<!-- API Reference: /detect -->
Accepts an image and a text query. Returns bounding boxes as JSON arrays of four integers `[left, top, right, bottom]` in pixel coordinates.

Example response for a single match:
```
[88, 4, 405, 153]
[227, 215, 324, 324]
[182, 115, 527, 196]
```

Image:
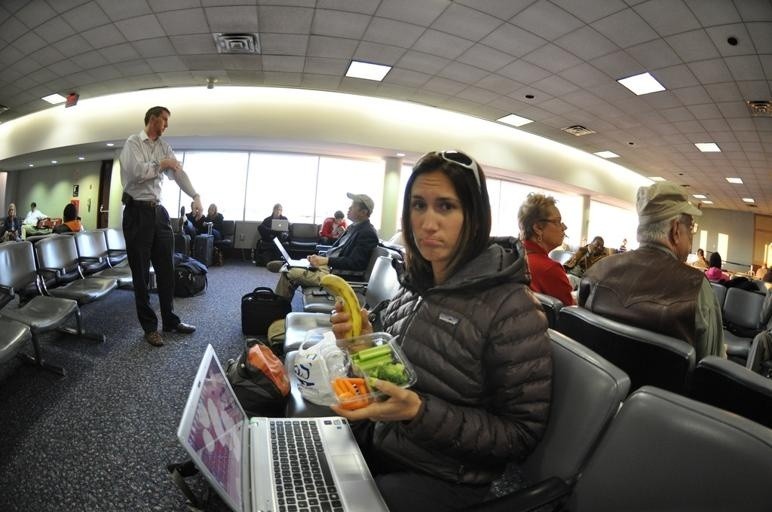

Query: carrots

[332, 376, 369, 408]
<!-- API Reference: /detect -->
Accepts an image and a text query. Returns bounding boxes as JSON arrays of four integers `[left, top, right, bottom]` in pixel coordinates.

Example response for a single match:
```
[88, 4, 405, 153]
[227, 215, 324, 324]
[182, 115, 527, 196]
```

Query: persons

[266, 192, 380, 303]
[514, 193, 577, 306]
[21, 201, 49, 240]
[51, 203, 82, 234]
[178, 201, 205, 247]
[332, 147, 555, 511]
[318, 210, 348, 247]
[202, 203, 225, 266]
[2, 202, 23, 242]
[257, 202, 293, 246]
[119, 105, 205, 347]
[690, 248, 729, 287]
[563, 235, 629, 279]
[577, 180, 729, 364]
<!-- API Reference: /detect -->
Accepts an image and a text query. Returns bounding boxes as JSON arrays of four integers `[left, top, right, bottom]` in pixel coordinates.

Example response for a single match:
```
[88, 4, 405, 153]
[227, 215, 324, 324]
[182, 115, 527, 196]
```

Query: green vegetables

[352, 342, 393, 369]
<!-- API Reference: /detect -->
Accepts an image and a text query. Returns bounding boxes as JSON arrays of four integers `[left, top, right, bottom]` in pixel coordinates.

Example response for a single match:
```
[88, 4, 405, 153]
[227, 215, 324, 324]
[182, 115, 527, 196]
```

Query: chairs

[218, 220, 233, 264]
[75, 228, 134, 292]
[290, 222, 317, 258]
[1, 240, 81, 381]
[38, 231, 118, 345]
[1, 281, 33, 392]
[109, 227, 154, 279]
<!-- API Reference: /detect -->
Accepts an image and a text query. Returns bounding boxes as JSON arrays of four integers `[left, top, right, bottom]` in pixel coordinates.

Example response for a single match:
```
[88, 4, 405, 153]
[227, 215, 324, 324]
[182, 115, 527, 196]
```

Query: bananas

[320, 274, 362, 339]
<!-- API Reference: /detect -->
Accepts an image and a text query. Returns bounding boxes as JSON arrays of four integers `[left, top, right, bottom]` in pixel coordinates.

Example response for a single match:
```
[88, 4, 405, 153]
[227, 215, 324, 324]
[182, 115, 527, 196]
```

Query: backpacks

[172, 252, 209, 299]
[224, 338, 292, 416]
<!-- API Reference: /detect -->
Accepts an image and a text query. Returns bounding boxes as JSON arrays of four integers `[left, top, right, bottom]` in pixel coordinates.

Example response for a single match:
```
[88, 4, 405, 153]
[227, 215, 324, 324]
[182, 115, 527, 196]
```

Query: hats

[636, 180, 703, 225]
[8, 203, 15, 210]
[347, 192, 374, 213]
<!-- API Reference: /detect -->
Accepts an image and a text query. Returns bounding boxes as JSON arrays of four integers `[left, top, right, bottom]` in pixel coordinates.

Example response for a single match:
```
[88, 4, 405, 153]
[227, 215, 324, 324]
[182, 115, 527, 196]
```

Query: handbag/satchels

[255, 238, 265, 267]
[241, 285, 293, 337]
[37, 219, 48, 229]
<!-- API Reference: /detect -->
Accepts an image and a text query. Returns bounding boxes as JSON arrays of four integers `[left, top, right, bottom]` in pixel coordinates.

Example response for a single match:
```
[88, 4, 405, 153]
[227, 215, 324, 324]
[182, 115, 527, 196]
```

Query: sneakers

[281, 233, 288, 239]
[266, 260, 287, 273]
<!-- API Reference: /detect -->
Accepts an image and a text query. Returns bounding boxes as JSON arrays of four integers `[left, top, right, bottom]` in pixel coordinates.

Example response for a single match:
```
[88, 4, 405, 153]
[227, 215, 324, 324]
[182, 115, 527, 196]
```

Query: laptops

[177, 343, 391, 512]
[271, 219, 289, 233]
[272, 236, 320, 271]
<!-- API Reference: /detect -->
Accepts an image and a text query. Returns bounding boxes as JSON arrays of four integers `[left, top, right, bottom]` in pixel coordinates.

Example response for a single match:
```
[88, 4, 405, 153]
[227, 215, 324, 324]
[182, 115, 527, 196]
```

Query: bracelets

[191, 193, 201, 199]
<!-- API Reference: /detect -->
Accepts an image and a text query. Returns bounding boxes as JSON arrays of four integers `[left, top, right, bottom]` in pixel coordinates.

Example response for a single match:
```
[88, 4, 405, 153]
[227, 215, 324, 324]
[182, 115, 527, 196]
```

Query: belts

[126, 201, 156, 207]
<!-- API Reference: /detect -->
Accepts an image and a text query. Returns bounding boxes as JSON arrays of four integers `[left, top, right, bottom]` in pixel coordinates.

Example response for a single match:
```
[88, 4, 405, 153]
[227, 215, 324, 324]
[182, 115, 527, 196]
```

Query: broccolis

[375, 363, 407, 383]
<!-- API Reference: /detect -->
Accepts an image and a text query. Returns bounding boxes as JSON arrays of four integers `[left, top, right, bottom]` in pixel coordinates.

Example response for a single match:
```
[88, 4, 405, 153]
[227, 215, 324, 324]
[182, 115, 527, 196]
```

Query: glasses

[413, 149, 482, 195]
[679, 221, 698, 233]
[536, 219, 560, 226]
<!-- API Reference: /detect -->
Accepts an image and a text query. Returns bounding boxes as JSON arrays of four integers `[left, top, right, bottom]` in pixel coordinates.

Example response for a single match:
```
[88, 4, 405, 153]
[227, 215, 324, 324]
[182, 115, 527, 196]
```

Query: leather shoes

[145, 330, 164, 346]
[180, 206, 187, 223]
[162, 323, 197, 334]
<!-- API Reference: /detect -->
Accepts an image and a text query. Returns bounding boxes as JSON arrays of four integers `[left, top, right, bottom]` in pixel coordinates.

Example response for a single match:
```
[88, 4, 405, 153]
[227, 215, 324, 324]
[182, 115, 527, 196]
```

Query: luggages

[174, 221, 214, 267]
